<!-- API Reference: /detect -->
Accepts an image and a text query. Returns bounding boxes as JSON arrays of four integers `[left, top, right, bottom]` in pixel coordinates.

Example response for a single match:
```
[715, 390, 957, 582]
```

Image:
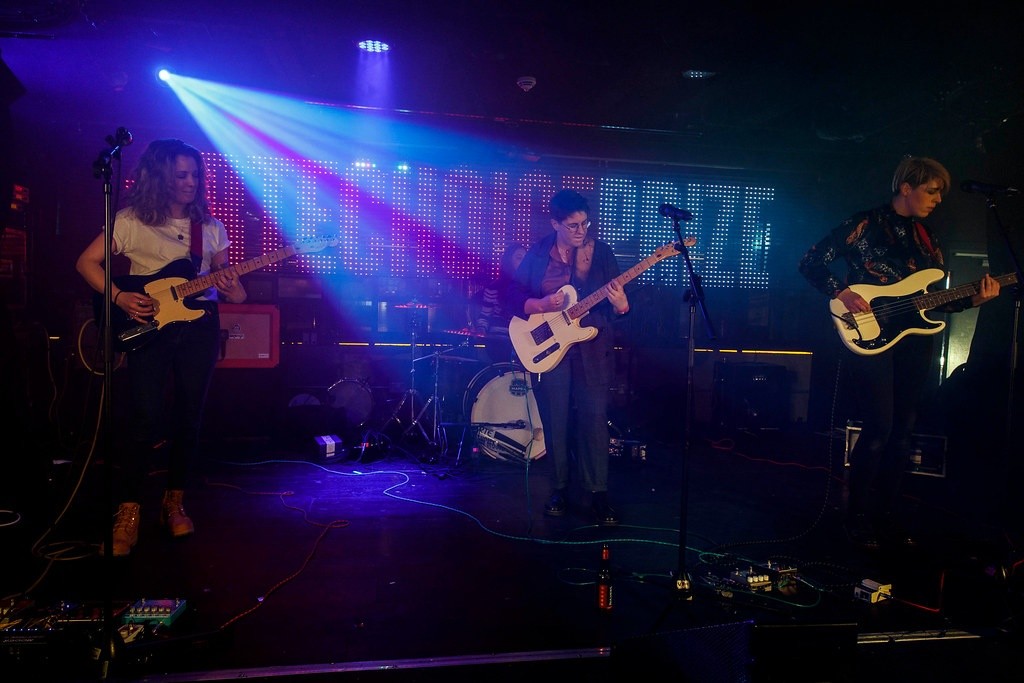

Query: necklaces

[167, 209, 188, 240]
[558, 241, 573, 254]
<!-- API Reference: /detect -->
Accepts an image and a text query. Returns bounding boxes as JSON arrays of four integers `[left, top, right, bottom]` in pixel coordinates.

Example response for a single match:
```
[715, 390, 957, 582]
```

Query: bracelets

[114, 290, 123, 304]
[617, 305, 629, 313]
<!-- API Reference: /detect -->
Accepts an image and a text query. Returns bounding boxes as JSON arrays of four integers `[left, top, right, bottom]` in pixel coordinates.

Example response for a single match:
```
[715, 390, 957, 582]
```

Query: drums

[461, 361, 547, 466]
[326, 376, 376, 425]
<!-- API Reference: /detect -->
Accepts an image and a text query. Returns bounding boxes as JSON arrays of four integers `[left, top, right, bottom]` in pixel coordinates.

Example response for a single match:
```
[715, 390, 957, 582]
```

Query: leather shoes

[601, 507, 619, 526]
[543, 490, 571, 517]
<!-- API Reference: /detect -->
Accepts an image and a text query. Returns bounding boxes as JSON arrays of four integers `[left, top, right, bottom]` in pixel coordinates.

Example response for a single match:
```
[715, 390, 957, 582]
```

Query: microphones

[116, 128, 132, 146]
[659, 204, 693, 221]
[220, 328, 229, 359]
[960, 179, 1020, 196]
[516, 420, 526, 429]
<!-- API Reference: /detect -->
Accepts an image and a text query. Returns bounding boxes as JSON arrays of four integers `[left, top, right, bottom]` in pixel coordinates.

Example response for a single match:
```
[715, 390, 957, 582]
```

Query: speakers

[215, 303, 281, 368]
[692, 348, 813, 426]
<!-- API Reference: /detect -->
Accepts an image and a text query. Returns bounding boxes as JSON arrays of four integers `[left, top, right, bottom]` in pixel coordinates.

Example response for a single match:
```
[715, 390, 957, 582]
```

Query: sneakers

[161, 489, 195, 537]
[100, 501, 141, 559]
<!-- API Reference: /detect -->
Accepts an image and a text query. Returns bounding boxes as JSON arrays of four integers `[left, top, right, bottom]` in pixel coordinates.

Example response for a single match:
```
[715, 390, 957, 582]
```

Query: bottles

[595, 543, 616, 612]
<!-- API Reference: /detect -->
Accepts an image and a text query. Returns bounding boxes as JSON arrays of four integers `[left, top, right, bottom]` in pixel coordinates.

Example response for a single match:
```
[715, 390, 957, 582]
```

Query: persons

[76, 137, 247, 557]
[799, 156, 1000, 549]
[475, 241, 528, 338]
[508, 187, 633, 529]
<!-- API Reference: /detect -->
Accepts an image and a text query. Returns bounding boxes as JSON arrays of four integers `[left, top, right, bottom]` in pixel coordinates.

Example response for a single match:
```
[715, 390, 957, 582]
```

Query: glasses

[561, 219, 592, 233]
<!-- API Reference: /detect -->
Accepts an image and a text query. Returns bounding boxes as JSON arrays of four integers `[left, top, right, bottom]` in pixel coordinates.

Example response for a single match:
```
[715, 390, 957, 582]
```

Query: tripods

[381, 307, 471, 456]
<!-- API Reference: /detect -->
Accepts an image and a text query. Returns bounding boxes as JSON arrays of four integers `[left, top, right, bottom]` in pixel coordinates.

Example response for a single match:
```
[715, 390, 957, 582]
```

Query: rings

[137, 299, 142, 305]
[134, 311, 138, 317]
[227, 284, 232, 288]
[131, 315, 134, 319]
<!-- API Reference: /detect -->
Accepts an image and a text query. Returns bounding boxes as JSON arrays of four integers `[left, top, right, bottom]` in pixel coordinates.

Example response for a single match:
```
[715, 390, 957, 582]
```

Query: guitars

[508, 234, 696, 375]
[826, 267, 1024, 358]
[93, 233, 339, 356]
